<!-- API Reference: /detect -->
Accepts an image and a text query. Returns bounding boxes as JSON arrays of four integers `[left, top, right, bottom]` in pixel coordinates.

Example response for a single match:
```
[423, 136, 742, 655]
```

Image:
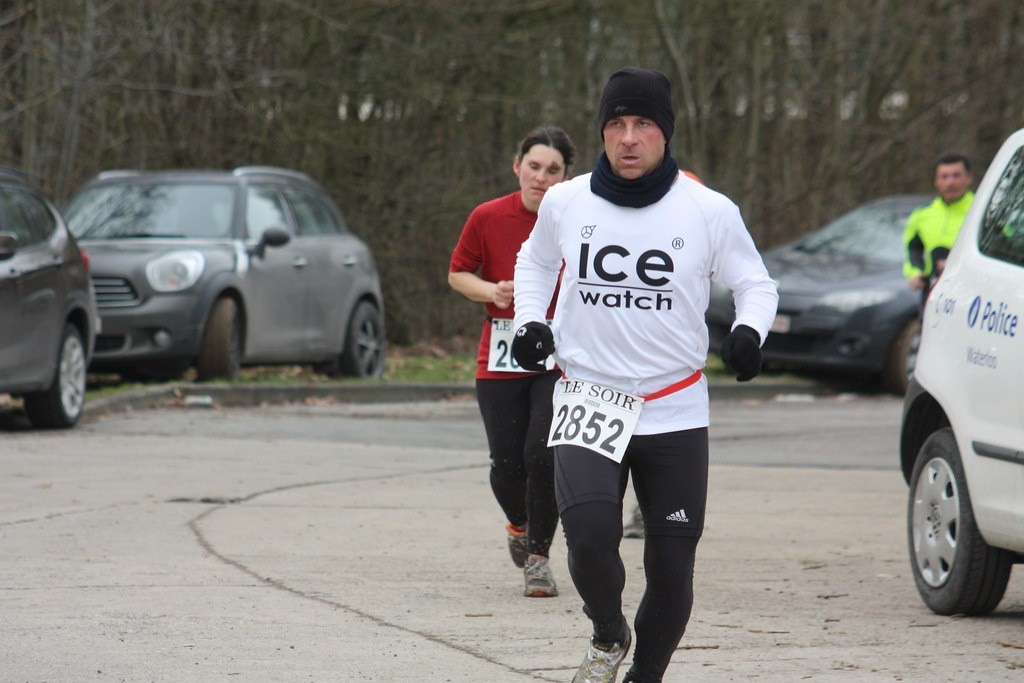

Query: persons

[513, 67, 780, 683]
[448, 125, 565, 598]
[620, 496, 647, 538]
[902, 152, 977, 314]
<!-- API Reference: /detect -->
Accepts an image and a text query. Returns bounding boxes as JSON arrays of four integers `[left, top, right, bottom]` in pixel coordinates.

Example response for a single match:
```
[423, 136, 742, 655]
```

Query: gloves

[718, 324, 762, 381]
[510, 321, 555, 372]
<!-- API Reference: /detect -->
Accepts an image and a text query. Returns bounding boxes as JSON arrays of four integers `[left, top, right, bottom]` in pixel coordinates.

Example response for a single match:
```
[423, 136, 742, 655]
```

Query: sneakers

[504, 522, 530, 568]
[623, 506, 645, 537]
[572, 614, 632, 683]
[523, 551, 559, 596]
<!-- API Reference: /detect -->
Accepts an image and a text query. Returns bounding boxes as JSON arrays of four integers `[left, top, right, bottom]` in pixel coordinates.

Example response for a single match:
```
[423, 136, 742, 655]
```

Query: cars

[897, 130, 1023, 617]
[705, 194, 941, 396]
[70, 166, 388, 384]
[1, 174, 99, 429]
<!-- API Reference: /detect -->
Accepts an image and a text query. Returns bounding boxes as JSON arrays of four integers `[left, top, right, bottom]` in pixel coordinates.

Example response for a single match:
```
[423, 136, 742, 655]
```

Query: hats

[598, 67, 676, 145]
